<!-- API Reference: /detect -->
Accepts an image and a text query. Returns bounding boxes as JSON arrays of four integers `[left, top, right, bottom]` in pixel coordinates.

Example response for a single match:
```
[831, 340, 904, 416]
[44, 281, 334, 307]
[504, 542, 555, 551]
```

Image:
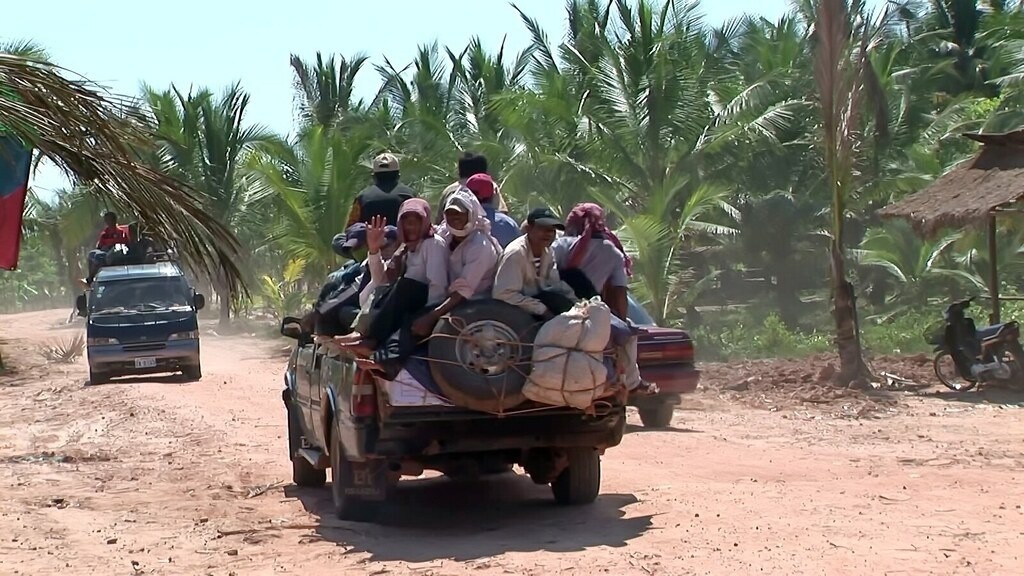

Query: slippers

[630, 380, 661, 397]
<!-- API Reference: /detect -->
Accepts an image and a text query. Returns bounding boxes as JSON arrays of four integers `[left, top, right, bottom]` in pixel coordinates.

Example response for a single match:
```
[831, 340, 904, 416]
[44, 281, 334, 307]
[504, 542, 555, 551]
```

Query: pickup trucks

[281, 261, 630, 521]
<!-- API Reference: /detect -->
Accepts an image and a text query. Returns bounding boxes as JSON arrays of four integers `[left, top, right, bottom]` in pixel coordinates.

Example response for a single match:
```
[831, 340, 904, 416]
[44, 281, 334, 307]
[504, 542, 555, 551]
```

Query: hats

[443, 203, 467, 213]
[342, 222, 367, 248]
[527, 208, 565, 231]
[373, 153, 400, 172]
[467, 172, 494, 199]
[380, 225, 397, 251]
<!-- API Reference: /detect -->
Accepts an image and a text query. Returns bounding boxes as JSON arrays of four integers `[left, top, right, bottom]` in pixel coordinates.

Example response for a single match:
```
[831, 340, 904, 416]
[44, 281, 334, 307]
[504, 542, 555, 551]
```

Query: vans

[76, 252, 205, 385]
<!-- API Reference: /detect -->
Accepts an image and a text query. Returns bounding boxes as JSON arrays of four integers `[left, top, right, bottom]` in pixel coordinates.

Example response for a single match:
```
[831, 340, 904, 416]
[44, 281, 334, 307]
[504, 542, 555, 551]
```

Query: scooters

[922, 295, 1024, 391]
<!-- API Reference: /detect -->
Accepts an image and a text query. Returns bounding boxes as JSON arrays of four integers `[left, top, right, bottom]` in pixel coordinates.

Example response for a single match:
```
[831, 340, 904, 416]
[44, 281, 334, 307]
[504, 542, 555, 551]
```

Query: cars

[625, 292, 702, 429]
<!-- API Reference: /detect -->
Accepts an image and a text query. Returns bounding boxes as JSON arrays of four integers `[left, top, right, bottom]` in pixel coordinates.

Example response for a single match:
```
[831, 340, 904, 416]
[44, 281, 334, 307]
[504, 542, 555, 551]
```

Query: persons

[341, 154, 418, 230]
[300, 219, 402, 343]
[158, 280, 186, 305]
[493, 207, 578, 323]
[339, 186, 506, 381]
[434, 153, 566, 249]
[551, 203, 661, 396]
[334, 194, 448, 345]
[86, 211, 130, 283]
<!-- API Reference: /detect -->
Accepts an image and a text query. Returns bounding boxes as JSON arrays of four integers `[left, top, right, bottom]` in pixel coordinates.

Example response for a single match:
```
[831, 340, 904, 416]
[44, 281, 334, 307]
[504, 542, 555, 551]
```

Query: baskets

[923, 318, 947, 345]
